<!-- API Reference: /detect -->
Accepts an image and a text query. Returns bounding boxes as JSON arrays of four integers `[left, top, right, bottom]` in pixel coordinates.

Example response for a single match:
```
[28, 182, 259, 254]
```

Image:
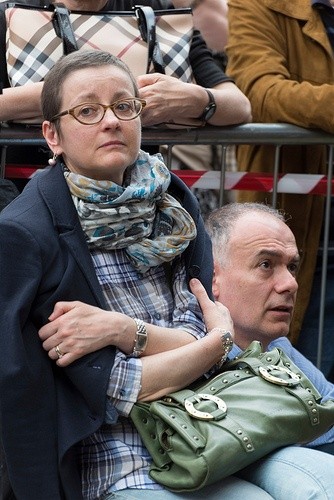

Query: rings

[56, 346, 63, 358]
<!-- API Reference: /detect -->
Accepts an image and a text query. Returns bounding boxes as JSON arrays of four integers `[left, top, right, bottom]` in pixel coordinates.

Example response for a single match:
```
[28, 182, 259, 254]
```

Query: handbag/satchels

[4, 2, 207, 129]
[132, 338, 334, 494]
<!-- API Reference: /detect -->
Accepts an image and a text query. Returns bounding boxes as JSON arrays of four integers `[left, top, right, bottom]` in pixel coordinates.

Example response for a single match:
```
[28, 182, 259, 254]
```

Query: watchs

[198, 90, 216, 121]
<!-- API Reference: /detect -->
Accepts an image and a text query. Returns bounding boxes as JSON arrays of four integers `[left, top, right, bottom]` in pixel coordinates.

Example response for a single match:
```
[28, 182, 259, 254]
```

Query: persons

[0, 51, 334, 500]
[205, 202, 334, 456]
[0, 0, 334, 385]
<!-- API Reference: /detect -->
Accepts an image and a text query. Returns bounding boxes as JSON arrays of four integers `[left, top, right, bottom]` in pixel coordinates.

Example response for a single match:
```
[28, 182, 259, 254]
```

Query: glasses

[49, 97, 146, 125]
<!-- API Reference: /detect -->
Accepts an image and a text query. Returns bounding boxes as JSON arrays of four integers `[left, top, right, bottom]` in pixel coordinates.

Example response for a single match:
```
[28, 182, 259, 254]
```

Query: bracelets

[206, 327, 233, 374]
[126, 318, 148, 358]
